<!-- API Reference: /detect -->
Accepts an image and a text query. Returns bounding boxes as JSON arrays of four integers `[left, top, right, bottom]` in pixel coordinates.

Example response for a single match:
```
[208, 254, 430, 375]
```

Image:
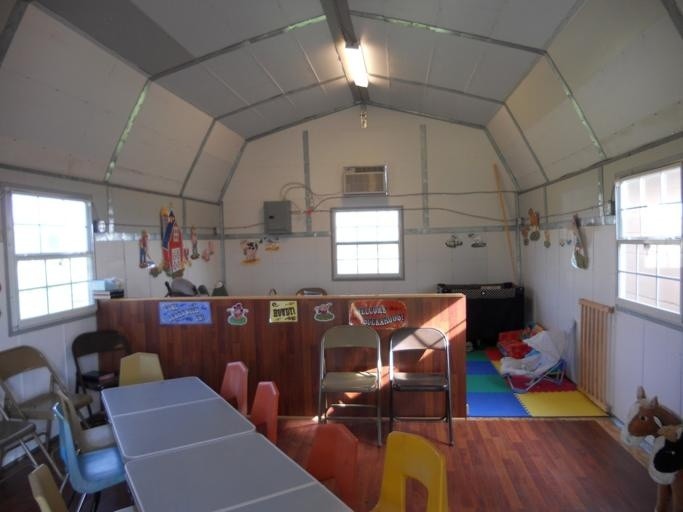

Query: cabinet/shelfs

[437, 282, 525, 349]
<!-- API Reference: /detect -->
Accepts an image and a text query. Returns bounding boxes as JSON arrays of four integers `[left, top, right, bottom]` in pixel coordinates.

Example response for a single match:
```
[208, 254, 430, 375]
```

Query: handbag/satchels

[571, 216, 587, 269]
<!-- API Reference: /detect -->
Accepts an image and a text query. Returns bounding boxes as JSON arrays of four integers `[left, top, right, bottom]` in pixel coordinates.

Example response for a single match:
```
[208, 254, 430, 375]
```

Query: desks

[124, 432, 355, 512]
[101, 375, 256, 461]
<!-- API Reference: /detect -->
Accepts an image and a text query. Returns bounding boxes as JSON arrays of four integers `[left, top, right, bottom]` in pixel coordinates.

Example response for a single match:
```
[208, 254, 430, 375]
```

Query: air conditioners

[343, 165, 388, 196]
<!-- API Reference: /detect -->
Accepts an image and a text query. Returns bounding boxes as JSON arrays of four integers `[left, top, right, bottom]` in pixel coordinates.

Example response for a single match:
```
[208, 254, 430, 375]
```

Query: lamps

[345, 43, 368, 88]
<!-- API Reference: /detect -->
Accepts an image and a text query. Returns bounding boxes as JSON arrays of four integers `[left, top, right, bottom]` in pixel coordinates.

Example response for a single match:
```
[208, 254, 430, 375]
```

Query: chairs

[28, 463, 140, 511]
[220, 360, 248, 415]
[57, 387, 115, 510]
[0, 345, 93, 480]
[119, 352, 164, 386]
[52, 402, 126, 512]
[369, 432, 449, 512]
[318, 324, 382, 447]
[0, 406, 38, 468]
[72, 330, 134, 427]
[250, 381, 279, 444]
[389, 327, 453, 446]
[306, 424, 359, 508]
